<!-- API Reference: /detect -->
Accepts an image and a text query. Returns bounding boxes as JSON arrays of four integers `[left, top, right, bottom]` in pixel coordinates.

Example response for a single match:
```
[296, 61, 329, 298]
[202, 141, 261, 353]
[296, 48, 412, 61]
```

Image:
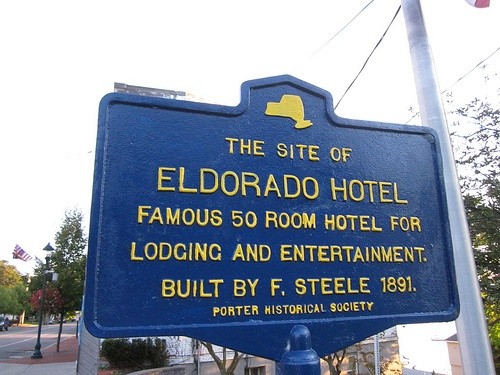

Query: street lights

[26, 242, 55, 361]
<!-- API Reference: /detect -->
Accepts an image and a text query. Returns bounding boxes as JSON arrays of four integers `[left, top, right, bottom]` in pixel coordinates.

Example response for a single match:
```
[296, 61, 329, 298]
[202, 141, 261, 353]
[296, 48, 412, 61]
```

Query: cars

[0, 316, 9, 332]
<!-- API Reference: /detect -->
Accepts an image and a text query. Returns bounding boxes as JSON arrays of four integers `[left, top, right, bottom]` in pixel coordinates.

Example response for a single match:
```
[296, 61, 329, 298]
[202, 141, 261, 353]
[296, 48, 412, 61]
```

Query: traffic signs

[80, 72, 462, 340]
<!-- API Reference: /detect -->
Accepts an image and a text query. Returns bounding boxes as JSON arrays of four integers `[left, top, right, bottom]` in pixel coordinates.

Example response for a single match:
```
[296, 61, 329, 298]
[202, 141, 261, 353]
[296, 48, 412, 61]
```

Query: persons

[3, 317, 9, 331]
